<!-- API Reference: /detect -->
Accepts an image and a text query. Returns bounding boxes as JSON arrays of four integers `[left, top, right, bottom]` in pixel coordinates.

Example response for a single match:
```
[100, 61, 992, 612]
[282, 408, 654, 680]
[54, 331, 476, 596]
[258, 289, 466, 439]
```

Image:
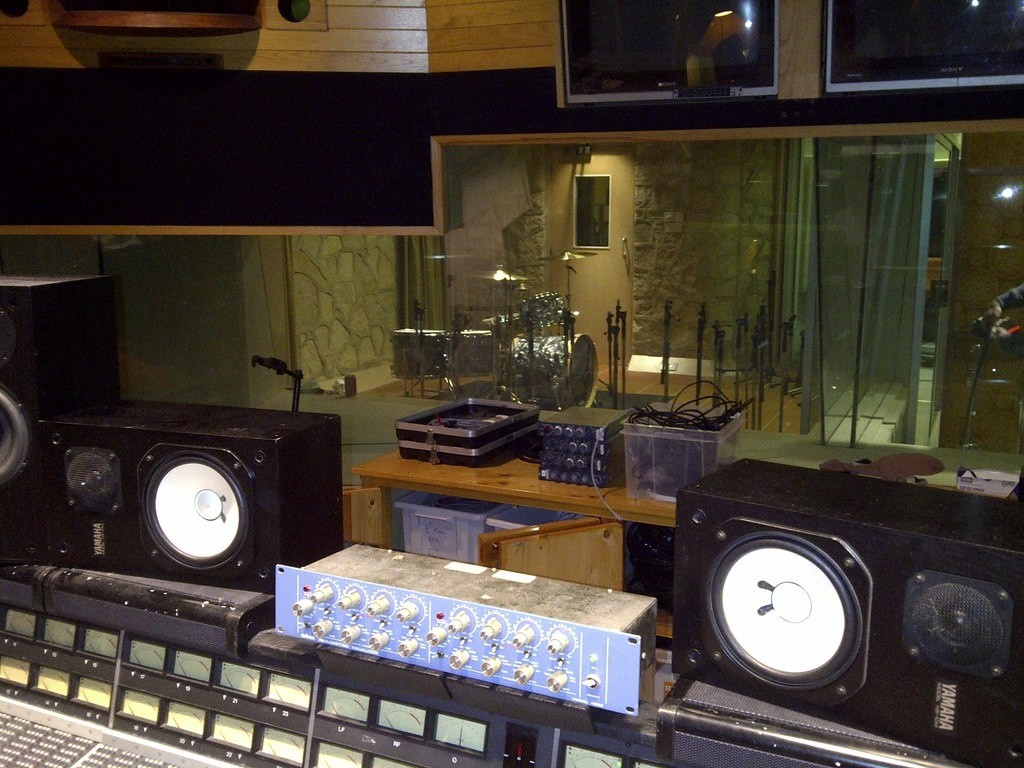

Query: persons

[981, 281, 1024, 359]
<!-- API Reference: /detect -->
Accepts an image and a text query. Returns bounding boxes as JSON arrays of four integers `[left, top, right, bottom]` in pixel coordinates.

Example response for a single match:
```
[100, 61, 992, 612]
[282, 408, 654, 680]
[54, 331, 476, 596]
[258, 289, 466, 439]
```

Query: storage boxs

[395, 397, 540, 467]
[393, 491, 512, 565]
[619, 401, 745, 502]
[485, 506, 584, 531]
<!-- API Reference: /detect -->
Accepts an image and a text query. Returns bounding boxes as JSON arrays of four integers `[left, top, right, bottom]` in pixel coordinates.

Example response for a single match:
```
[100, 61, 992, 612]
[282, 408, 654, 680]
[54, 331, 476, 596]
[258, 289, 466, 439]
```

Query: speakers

[0, 272, 342, 596]
[673, 458, 1024, 768]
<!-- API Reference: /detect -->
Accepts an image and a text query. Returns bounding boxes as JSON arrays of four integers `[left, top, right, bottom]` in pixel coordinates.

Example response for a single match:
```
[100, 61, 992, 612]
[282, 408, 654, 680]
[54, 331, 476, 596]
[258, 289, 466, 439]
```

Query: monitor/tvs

[557, 0, 779, 110]
[820, 0, 1024, 98]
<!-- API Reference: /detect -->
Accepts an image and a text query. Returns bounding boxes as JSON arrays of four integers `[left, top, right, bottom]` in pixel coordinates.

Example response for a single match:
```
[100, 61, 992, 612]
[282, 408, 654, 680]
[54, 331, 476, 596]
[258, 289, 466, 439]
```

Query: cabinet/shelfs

[342, 448, 677, 640]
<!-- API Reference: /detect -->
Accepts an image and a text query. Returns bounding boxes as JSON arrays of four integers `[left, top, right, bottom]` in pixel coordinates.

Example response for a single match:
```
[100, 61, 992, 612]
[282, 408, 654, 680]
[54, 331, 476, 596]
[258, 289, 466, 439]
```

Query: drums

[502, 331, 598, 412]
[450, 330, 493, 376]
[390, 328, 450, 380]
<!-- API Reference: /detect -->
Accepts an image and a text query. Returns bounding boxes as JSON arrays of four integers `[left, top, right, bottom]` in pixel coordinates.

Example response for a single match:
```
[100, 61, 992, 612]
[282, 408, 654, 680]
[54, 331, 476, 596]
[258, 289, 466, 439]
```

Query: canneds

[344, 373, 357, 397]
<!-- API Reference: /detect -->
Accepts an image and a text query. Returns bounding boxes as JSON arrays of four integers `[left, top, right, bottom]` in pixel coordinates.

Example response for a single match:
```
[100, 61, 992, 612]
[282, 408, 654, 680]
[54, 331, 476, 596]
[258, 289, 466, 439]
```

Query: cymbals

[481, 313, 520, 323]
[471, 269, 530, 282]
[538, 252, 598, 261]
